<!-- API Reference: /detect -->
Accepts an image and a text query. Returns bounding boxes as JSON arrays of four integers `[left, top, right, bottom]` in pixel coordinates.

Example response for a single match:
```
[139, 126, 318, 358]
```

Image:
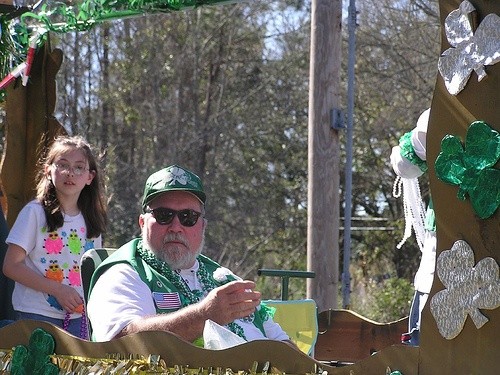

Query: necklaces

[63, 296, 87, 340]
[392, 175, 426, 253]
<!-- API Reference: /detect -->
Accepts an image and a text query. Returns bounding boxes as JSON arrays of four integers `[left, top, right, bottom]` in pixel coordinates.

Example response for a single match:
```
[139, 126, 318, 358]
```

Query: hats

[141, 164, 206, 209]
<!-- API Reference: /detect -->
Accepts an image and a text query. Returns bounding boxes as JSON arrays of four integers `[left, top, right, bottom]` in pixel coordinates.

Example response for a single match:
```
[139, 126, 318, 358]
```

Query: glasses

[145, 207, 202, 227]
[52, 164, 90, 176]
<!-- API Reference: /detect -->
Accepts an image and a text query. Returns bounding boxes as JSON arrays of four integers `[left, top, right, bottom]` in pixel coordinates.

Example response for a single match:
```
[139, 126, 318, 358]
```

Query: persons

[86, 164, 299, 351]
[3, 134, 110, 343]
[389, 106, 430, 344]
